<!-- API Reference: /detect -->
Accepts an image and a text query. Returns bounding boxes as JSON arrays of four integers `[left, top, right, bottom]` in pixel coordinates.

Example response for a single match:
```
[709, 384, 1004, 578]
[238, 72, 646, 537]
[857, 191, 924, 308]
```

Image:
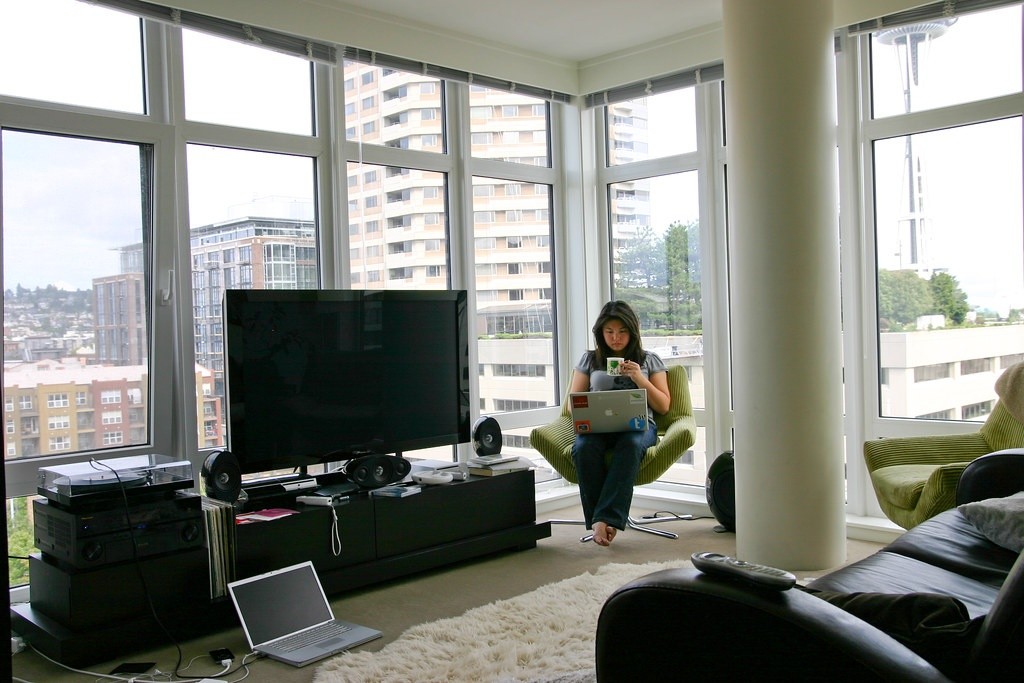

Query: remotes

[690, 550, 797, 590]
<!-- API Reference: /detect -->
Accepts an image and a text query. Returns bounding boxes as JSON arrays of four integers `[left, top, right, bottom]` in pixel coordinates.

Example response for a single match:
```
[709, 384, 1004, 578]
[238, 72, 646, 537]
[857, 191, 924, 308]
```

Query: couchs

[595, 447, 1024, 683]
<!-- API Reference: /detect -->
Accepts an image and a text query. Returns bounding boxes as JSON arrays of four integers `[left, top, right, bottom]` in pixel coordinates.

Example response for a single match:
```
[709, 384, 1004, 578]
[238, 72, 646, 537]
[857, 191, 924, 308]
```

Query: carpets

[312, 557, 697, 683]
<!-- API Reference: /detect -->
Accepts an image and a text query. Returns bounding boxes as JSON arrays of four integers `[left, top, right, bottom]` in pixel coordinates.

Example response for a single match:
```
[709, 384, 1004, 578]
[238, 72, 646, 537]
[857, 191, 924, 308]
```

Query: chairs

[861, 362, 1024, 530]
[531, 364, 695, 543]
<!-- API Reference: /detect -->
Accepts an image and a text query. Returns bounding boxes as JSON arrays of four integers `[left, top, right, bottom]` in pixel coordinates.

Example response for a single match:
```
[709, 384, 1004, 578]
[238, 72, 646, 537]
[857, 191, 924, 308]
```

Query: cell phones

[209, 648, 236, 664]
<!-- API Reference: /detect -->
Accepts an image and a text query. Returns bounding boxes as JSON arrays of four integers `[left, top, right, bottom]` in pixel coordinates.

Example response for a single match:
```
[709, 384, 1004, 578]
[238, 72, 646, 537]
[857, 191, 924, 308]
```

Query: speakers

[201, 450, 241, 504]
[472, 415, 503, 458]
[344, 455, 411, 490]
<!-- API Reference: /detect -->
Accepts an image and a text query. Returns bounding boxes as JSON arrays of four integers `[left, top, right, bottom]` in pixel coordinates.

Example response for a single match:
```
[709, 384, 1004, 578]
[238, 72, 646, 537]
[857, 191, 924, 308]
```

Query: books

[368, 486, 421, 497]
[467, 454, 537, 476]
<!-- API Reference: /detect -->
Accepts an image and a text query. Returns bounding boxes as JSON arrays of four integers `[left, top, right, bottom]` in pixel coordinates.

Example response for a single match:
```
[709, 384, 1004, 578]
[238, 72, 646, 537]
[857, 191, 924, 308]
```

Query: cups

[607, 357, 630, 376]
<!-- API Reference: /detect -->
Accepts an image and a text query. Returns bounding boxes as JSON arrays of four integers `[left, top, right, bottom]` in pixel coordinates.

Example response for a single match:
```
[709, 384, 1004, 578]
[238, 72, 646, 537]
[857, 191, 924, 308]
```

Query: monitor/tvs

[222, 289, 471, 497]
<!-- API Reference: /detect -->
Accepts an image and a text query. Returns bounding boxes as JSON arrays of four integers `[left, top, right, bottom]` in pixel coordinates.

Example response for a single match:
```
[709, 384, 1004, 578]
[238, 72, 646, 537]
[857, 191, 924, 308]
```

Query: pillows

[958, 490, 1024, 554]
[809, 593, 969, 675]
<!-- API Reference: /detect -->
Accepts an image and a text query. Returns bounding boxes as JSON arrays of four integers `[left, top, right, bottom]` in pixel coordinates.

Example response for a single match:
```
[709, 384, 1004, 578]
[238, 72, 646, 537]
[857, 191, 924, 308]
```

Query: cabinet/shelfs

[10, 465, 550, 670]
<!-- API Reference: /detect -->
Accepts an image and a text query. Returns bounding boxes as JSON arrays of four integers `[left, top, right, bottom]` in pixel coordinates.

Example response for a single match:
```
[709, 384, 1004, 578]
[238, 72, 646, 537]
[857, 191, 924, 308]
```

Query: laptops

[229, 560, 383, 669]
[569, 388, 649, 434]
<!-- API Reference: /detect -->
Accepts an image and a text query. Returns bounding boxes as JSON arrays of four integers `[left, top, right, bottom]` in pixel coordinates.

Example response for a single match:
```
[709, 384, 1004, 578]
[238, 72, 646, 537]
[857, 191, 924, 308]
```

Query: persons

[567, 300, 671, 546]
